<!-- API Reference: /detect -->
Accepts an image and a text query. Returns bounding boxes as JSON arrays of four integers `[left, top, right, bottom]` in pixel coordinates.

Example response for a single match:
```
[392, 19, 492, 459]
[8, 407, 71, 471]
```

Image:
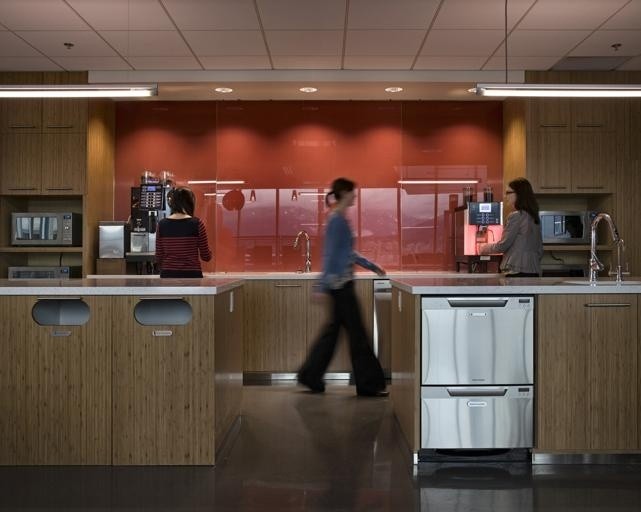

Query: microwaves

[539, 211, 600, 244]
[8, 265, 82, 280]
[11, 212, 81, 245]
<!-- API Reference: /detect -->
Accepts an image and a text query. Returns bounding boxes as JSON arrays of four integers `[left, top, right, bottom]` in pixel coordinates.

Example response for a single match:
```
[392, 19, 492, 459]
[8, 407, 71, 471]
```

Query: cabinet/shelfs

[1, 72, 118, 277]
[534, 295, 641, 458]
[3, 288, 244, 464]
[246, 280, 374, 372]
[501, 67, 641, 273]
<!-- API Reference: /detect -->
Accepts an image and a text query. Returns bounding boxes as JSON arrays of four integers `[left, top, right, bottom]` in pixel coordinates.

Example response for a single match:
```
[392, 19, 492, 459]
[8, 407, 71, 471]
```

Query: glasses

[504, 191, 516, 195]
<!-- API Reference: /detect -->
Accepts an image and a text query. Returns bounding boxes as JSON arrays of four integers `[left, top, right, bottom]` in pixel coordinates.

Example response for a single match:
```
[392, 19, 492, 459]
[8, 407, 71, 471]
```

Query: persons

[153, 186, 212, 278]
[297, 177, 393, 397]
[477, 177, 544, 278]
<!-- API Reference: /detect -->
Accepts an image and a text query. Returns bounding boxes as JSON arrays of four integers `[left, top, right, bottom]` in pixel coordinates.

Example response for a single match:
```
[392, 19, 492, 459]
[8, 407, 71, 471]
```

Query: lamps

[477, 0, 641, 104]
[290, 191, 297, 202]
[1, 1, 157, 102]
[248, 188, 257, 202]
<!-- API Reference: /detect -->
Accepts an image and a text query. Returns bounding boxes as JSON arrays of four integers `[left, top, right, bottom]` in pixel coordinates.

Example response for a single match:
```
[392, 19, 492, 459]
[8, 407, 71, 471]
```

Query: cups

[139, 169, 154, 184]
[157, 170, 176, 186]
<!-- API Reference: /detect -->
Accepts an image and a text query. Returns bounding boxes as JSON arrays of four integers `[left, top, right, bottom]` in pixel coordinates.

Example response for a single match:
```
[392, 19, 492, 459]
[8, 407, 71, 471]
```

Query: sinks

[562, 279, 641, 288]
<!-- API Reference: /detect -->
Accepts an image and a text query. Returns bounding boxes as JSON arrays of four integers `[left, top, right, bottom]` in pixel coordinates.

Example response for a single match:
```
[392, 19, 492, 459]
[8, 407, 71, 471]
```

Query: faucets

[292, 229, 312, 272]
[587, 208, 619, 281]
[617, 237, 625, 281]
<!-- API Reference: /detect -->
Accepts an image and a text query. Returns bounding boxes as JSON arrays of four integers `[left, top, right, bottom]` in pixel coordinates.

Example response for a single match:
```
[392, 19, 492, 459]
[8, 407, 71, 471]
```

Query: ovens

[419, 297, 532, 450]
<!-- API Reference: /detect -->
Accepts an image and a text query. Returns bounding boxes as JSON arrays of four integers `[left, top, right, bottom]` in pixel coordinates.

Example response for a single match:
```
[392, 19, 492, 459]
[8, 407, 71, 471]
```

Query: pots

[221, 188, 244, 211]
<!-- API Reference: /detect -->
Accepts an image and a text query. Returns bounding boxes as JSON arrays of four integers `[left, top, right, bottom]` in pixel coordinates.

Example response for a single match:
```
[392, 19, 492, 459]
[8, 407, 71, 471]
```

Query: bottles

[483, 183, 493, 202]
[448, 194, 458, 212]
[462, 183, 473, 205]
